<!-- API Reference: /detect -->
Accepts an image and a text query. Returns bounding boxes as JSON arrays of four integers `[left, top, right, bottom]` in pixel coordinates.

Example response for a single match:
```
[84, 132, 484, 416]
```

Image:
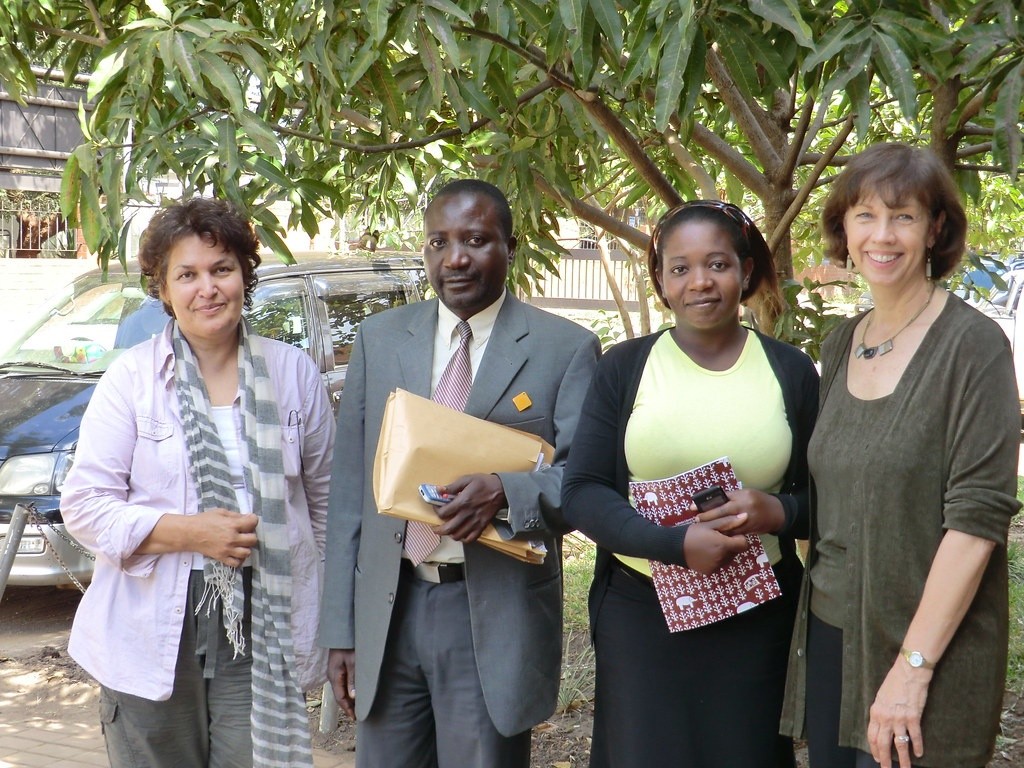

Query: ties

[403, 322, 474, 569]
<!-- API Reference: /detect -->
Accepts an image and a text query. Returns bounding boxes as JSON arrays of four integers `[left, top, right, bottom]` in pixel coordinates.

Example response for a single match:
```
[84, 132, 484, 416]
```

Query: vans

[1, 248, 433, 589]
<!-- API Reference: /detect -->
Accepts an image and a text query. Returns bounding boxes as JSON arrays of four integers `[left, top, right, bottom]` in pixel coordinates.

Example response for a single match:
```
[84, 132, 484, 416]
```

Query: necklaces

[854, 280, 936, 360]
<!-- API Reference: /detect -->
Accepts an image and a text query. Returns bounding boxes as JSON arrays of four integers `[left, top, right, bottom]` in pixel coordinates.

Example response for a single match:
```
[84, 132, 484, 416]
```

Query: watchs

[898, 646, 937, 670]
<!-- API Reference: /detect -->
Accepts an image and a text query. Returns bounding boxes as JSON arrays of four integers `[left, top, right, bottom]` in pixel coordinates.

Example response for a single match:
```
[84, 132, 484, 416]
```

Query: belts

[400, 558, 466, 585]
[608, 555, 805, 641]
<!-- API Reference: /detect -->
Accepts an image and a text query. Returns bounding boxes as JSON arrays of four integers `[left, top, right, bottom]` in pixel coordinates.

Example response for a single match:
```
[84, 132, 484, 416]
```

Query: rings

[893, 734, 910, 743]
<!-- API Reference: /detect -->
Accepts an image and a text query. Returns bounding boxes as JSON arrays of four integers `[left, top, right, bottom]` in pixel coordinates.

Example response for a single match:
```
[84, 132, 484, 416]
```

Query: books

[627, 456, 783, 634]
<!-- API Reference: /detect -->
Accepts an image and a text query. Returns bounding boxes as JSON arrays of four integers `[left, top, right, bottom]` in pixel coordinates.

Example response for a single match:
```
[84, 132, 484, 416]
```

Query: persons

[774, 142, 1023, 768]
[317, 177, 605, 768]
[559, 197, 826, 768]
[58, 197, 337, 768]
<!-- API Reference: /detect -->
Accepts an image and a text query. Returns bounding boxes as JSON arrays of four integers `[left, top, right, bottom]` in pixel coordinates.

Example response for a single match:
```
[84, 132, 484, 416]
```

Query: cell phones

[418, 484, 452, 506]
[691, 485, 730, 514]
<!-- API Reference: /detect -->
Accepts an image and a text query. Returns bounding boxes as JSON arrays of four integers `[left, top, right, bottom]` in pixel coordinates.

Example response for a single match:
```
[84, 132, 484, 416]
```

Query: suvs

[946, 251, 1024, 416]
